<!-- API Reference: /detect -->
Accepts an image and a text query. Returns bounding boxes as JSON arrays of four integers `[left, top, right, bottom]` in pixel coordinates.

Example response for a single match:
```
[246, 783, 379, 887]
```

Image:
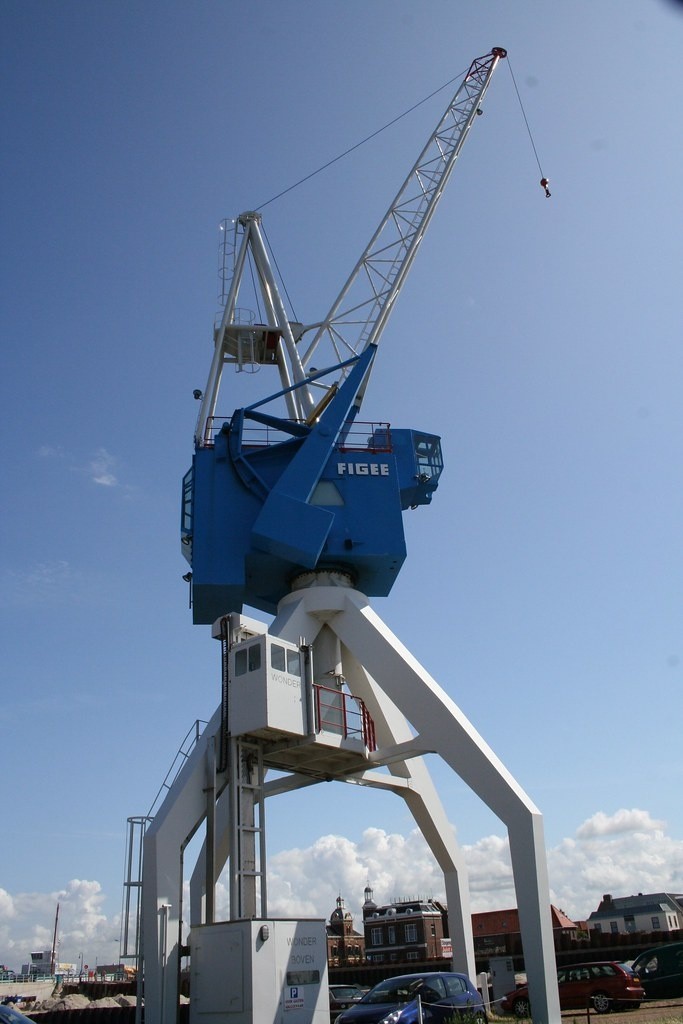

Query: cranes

[134, 47, 583, 1024]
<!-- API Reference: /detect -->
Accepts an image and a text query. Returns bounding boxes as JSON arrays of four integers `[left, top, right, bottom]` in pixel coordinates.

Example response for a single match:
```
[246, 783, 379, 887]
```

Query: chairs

[570, 972, 577, 980]
[580, 972, 588, 979]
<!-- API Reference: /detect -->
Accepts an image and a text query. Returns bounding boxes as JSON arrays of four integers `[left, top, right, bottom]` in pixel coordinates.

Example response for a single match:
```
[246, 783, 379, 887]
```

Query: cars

[0, 1004, 38, 1024]
[501, 961, 645, 1019]
[329, 985, 367, 1024]
[334, 971, 491, 1024]
[631, 941, 683, 999]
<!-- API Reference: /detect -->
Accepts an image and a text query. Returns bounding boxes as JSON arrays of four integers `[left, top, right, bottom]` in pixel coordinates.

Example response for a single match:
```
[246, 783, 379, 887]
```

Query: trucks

[0, 964, 15, 974]
[1, 994, 37, 1005]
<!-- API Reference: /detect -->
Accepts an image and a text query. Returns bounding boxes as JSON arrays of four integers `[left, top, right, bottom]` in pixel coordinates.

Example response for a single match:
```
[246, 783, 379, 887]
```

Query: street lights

[115, 939, 121, 973]
[79, 952, 84, 973]
